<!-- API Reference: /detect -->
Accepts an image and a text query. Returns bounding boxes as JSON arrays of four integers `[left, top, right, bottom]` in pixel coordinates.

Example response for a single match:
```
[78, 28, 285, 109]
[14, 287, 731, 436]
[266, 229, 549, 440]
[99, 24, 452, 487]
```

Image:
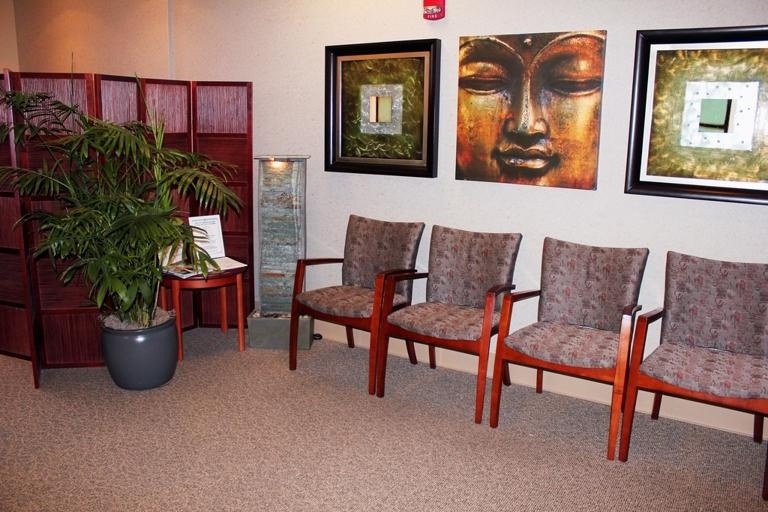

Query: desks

[159, 267, 245, 361]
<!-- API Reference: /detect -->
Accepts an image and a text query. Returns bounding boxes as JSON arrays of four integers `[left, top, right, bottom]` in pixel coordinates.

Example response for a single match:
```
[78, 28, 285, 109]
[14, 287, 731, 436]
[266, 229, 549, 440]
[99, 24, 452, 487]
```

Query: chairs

[618, 250, 768, 505]
[288, 215, 426, 396]
[490, 237, 650, 461]
[376, 224, 522, 423]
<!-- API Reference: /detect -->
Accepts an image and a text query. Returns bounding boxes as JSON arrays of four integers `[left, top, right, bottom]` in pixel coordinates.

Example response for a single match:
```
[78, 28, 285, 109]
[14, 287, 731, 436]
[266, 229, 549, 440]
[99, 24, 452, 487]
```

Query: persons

[455, 30, 608, 190]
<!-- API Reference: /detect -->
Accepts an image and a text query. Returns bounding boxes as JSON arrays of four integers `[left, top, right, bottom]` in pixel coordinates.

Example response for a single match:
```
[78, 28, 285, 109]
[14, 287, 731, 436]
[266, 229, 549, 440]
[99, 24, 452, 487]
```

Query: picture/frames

[625, 24, 768, 206]
[323, 38, 442, 178]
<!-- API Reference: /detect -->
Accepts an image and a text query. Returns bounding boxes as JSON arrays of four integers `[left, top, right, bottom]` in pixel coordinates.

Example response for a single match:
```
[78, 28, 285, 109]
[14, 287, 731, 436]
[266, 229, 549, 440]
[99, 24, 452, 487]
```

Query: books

[157, 240, 212, 279]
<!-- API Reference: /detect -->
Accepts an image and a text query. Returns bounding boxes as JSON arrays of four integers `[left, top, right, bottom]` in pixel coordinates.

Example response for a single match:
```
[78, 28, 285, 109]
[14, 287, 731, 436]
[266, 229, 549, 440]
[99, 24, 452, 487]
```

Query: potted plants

[0, 86, 246, 391]
